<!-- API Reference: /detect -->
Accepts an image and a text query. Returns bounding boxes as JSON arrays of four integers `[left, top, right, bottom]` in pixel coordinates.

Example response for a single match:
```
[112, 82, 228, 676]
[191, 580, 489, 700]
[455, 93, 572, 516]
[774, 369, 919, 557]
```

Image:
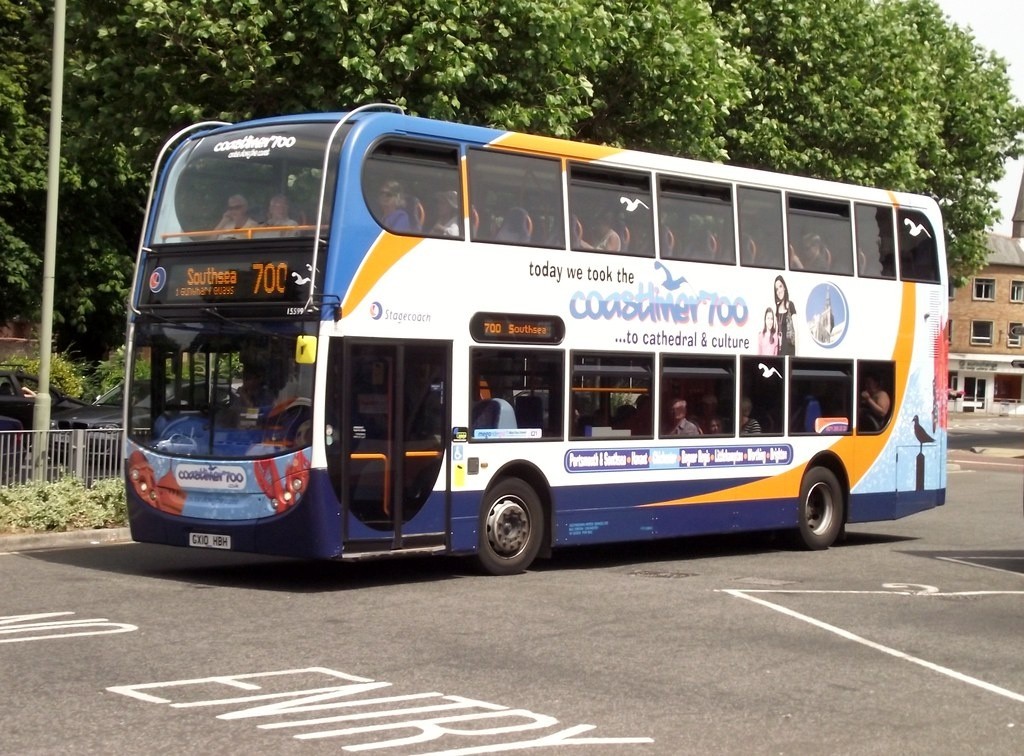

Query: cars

[0, 415, 25, 481]
[50, 378, 239, 469]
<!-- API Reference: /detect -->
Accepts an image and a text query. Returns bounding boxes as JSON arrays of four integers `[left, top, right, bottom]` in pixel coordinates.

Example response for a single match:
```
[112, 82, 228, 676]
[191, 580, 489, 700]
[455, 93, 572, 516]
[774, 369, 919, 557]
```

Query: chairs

[472, 401, 880, 433]
[291, 194, 867, 276]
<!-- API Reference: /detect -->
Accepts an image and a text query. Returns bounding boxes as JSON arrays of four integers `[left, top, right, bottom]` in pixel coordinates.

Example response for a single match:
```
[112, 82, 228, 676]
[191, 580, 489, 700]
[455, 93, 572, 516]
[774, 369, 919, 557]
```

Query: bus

[118, 102, 949, 576]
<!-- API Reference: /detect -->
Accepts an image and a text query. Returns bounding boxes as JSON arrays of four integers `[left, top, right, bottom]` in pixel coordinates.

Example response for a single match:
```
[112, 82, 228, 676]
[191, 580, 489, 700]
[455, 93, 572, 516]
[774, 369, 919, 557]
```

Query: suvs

[0, 362, 87, 452]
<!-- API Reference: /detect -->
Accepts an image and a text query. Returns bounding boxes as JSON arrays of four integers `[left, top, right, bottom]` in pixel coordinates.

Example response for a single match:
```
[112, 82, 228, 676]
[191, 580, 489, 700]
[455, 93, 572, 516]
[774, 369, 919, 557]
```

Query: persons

[740, 397, 762, 434]
[707, 416, 722, 433]
[593, 216, 622, 252]
[614, 394, 653, 437]
[252, 193, 301, 239]
[375, 179, 412, 231]
[427, 189, 460, 237]
[211, 194, 257, 240]
[758, 307, 779, 355]
[772, 274, 797, 357]
[667, 397, 699, 435]
[860, 370, 891, 426]
[798, 232, 827, 272]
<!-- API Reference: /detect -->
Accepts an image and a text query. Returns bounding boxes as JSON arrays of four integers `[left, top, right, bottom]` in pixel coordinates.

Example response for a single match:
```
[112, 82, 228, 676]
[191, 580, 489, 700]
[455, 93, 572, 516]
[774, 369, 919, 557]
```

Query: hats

[438, 190, 458, 209]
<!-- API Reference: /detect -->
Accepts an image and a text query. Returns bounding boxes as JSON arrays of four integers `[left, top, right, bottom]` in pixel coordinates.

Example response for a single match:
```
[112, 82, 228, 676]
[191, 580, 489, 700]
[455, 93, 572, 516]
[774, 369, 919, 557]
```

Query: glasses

[379, 191, 396, 197]
[228, 205, 244, 210]
